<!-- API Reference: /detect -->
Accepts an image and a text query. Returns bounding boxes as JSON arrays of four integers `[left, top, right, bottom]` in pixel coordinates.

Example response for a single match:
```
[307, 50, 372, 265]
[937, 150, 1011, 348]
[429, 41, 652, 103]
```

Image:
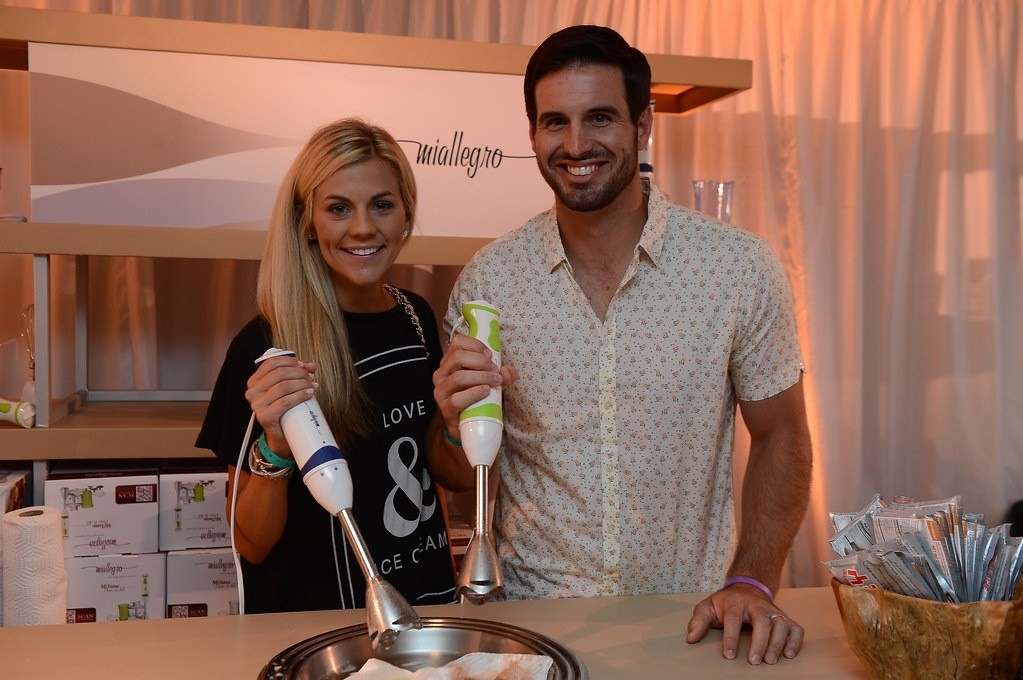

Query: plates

[255, 616, 587, 680]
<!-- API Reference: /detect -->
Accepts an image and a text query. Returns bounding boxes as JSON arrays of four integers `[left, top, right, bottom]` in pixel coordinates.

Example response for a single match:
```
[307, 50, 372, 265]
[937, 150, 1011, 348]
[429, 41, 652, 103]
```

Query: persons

[193, 118, 461, 614]
[432, 24, 815, 667]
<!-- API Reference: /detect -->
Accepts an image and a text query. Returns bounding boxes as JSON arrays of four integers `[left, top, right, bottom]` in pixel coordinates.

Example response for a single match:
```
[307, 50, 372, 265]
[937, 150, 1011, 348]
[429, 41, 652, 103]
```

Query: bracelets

[441, 420, 461, 447]
[247, 433, 293, 478]
[724, 576, 773, 602]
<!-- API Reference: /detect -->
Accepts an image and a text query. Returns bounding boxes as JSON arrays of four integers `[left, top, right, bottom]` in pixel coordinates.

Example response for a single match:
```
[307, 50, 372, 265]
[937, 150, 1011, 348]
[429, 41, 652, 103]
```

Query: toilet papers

[0, 506, 67, 627]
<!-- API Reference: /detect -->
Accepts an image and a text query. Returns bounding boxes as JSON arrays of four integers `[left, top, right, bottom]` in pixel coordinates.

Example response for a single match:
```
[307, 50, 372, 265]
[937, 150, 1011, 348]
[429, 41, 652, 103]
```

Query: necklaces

[383, 284, 430, 359]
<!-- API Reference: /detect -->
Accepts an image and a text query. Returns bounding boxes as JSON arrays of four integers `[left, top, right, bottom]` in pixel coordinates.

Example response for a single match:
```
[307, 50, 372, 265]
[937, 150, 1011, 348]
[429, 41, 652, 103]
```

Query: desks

[0, 587, 876, 680]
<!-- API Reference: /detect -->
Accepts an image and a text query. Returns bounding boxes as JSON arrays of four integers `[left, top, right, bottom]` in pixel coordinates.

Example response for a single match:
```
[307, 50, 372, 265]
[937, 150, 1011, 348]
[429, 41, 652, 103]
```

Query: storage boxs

[0, 467, 33, 515]
[44, 462, 240, 623]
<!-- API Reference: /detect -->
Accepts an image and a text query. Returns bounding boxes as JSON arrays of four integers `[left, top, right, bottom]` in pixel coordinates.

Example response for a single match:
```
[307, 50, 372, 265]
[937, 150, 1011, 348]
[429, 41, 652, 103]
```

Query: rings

[769, 614, 786, 620]
